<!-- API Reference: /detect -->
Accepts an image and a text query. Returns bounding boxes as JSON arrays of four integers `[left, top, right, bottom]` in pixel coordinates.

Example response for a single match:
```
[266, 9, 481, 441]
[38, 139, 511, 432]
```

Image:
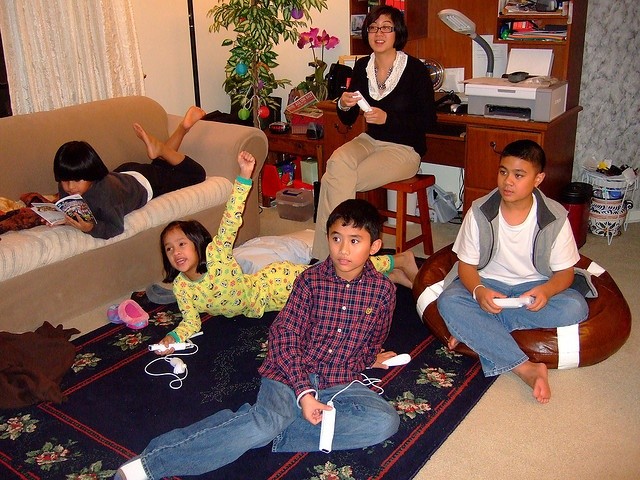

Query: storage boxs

[276, 188, 313, 222]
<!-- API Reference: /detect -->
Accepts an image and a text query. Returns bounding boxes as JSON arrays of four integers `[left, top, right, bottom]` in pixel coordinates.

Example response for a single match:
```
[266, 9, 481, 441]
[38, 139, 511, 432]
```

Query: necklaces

[372, 60, 395, 91]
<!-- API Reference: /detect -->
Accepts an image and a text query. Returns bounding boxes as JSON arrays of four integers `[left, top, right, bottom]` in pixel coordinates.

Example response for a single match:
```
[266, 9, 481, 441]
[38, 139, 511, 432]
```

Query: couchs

[1, 95, 269, 335]
[411, 243, 632, 369]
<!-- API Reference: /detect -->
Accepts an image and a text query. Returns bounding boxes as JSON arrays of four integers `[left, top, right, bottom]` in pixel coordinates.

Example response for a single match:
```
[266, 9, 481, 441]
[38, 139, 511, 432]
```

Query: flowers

[300, 27, 339, 113]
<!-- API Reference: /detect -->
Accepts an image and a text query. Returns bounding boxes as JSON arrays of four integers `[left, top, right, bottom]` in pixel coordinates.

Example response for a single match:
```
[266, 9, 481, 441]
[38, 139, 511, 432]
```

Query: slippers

[107, 299, 149, 329]
[131, 284, 177, 310]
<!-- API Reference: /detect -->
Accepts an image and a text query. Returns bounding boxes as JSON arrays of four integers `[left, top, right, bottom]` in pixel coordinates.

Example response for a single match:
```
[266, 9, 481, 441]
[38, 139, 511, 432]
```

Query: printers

[464, 76, 569, 122]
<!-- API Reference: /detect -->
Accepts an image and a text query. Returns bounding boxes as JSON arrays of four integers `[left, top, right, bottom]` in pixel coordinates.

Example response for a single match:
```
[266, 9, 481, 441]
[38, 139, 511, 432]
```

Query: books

[27, 199, 98, 228]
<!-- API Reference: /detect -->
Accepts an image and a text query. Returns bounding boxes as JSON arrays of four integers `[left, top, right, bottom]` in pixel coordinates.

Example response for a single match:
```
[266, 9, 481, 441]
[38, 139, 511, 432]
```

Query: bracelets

[470, 282, 486, 303]
[294, 387, 320, 412]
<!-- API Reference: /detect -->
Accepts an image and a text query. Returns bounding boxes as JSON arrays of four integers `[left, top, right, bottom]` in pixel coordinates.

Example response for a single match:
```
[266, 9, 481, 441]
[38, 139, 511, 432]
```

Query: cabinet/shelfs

[317, 100, 369, 214]
[350, 0, 429, 40]
[464, 117, 578, 237]
[493, 0, 589, 108]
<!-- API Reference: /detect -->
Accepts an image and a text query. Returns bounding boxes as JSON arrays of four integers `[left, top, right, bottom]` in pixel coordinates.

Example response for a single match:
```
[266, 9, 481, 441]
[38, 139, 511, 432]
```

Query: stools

[363, 173, 435, 257]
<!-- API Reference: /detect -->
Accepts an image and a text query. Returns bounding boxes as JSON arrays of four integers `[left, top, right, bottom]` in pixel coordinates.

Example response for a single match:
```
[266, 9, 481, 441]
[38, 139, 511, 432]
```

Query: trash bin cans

[556, 181, 593, 249]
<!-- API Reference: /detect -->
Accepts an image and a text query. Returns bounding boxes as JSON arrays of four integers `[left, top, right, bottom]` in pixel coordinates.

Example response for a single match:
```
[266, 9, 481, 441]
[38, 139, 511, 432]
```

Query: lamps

[439, 9, 494, 78]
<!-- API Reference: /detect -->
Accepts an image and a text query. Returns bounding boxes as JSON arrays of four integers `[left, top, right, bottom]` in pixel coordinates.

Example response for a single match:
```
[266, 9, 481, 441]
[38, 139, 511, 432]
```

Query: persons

[311, 4, 434, 268]
[436, 140, 590, 405]
[116, 197, 402, 480]
[52, 105, 212, 238]
[149, 149, 420, 353]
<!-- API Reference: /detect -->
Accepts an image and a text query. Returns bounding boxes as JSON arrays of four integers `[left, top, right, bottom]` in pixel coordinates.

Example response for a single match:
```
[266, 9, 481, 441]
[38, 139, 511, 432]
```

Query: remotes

[493, 296, 535, 310]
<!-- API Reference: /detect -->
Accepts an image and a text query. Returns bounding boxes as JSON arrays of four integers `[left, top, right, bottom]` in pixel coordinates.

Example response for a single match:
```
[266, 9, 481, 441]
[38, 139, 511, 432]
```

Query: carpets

[2, 250, 499, 478]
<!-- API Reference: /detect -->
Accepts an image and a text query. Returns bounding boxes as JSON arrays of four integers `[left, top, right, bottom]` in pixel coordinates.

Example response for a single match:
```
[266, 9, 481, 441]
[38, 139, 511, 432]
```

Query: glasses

[367, 26, 394, 33]
